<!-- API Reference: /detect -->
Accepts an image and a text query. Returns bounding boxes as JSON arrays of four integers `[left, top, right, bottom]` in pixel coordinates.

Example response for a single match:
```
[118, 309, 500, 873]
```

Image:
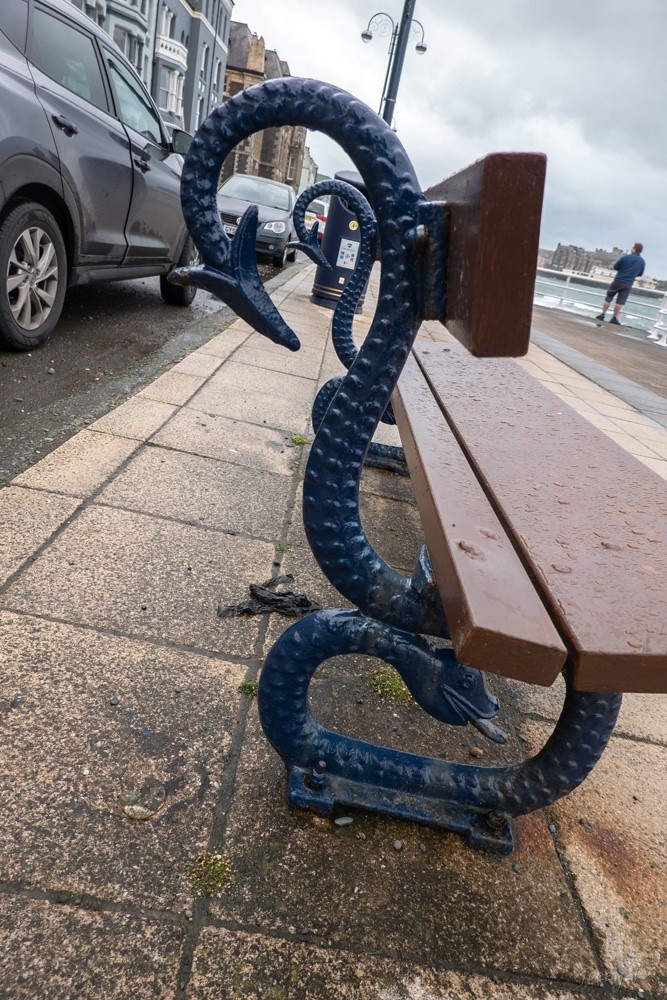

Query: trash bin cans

[310, 171, 375, 314]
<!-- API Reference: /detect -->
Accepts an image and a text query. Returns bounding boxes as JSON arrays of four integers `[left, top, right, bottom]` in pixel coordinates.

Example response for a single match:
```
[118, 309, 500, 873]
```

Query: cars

[297, 195, 330, 245]
[0, 0, 205, 353]
[215, 172, 300, 268]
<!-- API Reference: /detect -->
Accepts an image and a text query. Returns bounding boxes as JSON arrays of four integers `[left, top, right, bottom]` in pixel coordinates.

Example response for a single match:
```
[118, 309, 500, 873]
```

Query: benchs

[171, 76, 667, 854]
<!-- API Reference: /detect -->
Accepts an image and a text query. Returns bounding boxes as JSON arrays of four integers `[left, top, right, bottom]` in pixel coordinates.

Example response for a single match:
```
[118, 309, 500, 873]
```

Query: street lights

[361, 12, 427, 115]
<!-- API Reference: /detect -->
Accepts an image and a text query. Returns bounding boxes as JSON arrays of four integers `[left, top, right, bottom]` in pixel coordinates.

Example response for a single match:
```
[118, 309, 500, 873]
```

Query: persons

[596, 243, 645, 325]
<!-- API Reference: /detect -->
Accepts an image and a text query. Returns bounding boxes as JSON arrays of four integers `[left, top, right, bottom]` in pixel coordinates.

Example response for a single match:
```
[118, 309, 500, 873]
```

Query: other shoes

[596, 315, 604, 321]
[609, 319, 621, 325]
